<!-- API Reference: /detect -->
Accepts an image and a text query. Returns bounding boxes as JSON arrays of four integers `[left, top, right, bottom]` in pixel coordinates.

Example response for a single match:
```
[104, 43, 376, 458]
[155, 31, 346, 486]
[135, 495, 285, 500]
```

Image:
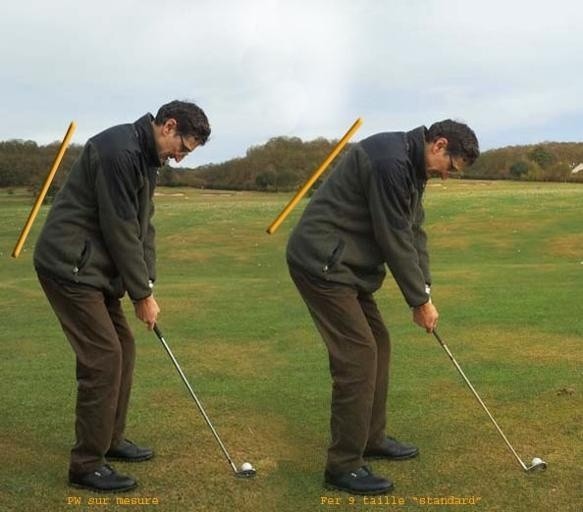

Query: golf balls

[240, 462, 253, 472]
[531, 457, 544, 466]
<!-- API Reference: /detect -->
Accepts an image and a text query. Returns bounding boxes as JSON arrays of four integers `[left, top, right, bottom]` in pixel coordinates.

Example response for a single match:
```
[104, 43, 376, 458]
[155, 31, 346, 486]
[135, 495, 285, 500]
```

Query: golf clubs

[153, 324, 256, 479]
[433, 328, 549, 474]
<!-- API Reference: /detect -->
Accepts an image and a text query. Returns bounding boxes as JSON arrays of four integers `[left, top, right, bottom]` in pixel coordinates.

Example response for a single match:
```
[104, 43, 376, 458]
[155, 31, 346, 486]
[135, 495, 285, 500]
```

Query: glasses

[176, 130, 193, 154]
[446, 150, 459, 173]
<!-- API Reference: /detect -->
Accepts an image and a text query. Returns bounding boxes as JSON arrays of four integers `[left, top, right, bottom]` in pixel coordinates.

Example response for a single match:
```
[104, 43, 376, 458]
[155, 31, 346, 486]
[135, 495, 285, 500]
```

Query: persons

[287, 119, 478, 494]
[33, 98, 212, 493]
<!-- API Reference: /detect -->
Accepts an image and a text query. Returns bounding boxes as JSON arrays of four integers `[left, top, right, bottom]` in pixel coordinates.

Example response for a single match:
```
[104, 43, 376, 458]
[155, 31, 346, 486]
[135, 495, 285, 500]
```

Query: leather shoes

[102, 438, 154, 463]
[320, 463, 395, 496]
[363, 436, 421, 461]
[66, 462, 138, 494]
